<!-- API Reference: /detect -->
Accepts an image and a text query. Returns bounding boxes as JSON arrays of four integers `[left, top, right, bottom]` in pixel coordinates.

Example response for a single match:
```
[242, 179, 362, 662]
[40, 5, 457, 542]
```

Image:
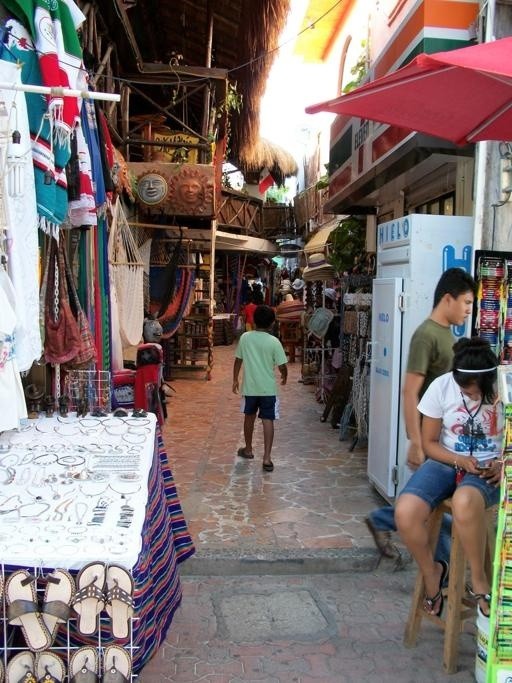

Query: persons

[232, 307, 289, 471]
[243, 283, 264, 332]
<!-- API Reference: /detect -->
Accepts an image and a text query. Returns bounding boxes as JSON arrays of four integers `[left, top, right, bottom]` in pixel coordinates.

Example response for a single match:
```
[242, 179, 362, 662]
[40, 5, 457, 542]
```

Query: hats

[301, 253, 335, 282]
[291, 278, 306, 290]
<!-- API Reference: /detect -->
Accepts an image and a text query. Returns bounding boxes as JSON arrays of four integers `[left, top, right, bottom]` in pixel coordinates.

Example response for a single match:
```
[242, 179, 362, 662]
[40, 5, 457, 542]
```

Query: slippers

[424, 559, 449, 618]
[363, 515, 401, 560]
[468, 588, 491, 618]
[262, 460, 273, 472]
[0, 561, 134, 683]
[238, 447, 254, 458]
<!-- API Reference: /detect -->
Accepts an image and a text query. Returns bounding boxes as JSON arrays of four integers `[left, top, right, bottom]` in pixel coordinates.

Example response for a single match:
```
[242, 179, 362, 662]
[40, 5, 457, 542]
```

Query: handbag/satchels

[43, 304, 98, 366]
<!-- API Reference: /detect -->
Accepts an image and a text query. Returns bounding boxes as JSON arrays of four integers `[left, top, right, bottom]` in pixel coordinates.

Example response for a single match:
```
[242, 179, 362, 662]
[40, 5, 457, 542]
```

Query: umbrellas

[305, 36, 512, 147]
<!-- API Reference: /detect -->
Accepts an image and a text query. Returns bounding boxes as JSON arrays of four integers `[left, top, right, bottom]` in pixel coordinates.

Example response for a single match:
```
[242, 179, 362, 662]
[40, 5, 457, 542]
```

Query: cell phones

[478, 467, 493, 469]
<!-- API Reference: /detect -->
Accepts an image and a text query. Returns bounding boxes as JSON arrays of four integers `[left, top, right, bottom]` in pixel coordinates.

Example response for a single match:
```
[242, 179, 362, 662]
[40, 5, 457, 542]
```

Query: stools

[403, 493, 497, 674]
